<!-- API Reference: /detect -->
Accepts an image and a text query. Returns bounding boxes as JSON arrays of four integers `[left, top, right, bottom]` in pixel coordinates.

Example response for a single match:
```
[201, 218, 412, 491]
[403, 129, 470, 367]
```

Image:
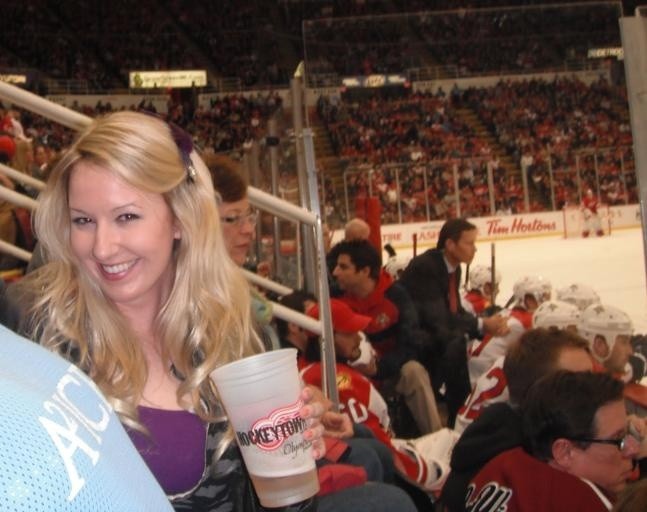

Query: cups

[208, 346, 321, 508]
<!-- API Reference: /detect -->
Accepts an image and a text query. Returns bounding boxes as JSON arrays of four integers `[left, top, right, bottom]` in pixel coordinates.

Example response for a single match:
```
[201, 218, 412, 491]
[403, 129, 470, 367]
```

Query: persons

[1, 2, 646, 510]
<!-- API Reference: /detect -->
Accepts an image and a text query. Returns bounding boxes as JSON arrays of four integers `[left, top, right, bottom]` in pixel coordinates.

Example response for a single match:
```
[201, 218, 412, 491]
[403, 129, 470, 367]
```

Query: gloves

[632, 336, 646, 383]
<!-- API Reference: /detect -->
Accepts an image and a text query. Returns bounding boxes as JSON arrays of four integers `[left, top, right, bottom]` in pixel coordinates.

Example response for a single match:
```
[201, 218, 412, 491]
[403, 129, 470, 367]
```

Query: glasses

[580, 419, 632, 450]
[216, 208, 258, 227]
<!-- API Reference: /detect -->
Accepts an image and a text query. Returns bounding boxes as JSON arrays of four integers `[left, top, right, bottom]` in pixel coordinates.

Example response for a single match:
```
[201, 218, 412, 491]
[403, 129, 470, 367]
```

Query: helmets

[513, 276, 631, 361]
[469, 266, 501, 300]
[383, 256, 413, 282]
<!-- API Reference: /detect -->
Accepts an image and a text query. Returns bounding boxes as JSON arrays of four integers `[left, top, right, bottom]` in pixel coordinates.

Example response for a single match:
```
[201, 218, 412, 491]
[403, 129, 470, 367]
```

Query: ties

[449, 271, 458, 314]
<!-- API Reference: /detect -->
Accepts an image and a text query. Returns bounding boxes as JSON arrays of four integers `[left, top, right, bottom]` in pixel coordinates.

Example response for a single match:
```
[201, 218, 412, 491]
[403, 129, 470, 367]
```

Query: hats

[0, 135, 17, 161]
[307, 299, 373, 336]
